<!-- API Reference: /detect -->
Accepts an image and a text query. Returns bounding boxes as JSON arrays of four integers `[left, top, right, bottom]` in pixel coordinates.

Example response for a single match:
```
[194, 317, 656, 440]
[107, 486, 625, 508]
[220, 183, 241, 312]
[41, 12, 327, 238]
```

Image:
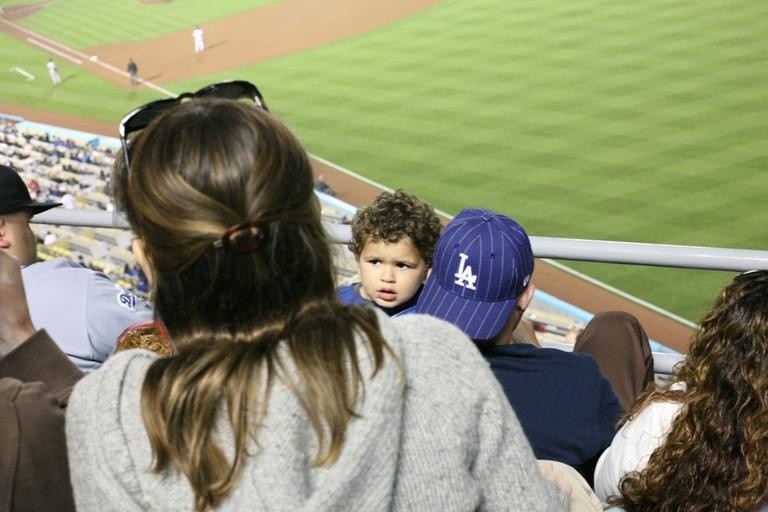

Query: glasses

[119, 81, 269, 174]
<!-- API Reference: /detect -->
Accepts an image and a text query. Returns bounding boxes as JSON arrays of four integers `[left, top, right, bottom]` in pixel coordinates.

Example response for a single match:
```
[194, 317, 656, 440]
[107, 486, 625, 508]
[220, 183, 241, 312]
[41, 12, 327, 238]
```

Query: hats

[417, 209, 534, 338]
[0, 164, 63, 216]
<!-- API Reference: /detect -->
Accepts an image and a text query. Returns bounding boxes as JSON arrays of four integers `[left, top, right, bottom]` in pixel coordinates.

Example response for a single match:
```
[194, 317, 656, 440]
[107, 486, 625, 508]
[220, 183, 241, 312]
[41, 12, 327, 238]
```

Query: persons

[192, 25, 205, 55]
[59, 94, 612, 512]
[333, 187, 444, 315]
[0, 164, 159, 376]
[587, 268, 768, 512]
[126, 57, 140, 86]
[0, 117, 151, 307]
[392, 207, 660, 491]
[45, 57, 62, 86]
[2, 248, 88, 510]
[313, 172, 336, 198]
